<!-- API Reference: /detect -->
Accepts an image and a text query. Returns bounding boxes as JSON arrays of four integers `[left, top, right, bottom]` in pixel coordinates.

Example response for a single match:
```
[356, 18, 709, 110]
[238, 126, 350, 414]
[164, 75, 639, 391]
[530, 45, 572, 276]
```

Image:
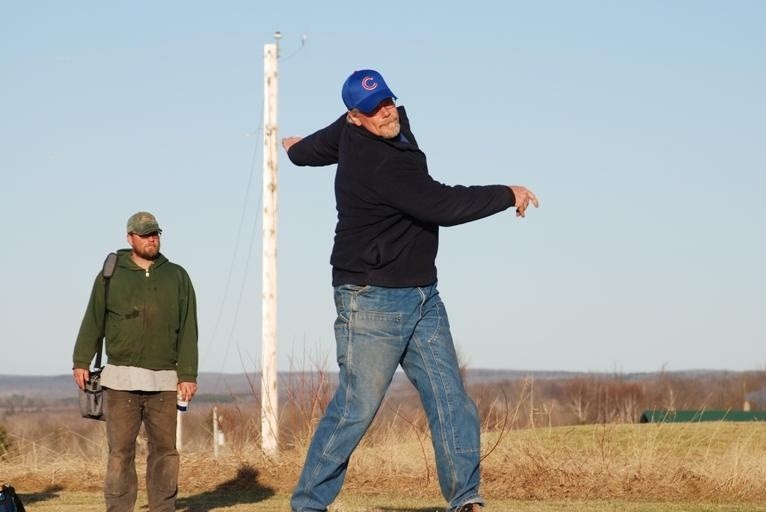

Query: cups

[176, 392, 188, 414]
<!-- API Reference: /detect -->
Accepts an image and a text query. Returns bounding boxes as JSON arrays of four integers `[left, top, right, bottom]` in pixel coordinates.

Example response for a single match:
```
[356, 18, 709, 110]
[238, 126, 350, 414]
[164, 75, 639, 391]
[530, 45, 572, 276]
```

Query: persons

[282, 71, 539, 512]
[69, 210, 198, 512]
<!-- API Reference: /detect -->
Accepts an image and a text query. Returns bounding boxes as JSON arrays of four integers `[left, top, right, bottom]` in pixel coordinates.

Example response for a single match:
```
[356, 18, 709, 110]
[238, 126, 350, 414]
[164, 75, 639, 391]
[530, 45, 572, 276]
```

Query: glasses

[132, 232, 162, 239]
[370, 96, 396, 114]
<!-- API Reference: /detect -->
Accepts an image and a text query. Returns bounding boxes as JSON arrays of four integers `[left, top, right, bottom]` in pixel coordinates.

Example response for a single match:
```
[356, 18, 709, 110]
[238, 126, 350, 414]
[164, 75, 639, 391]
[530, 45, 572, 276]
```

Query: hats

[341, 68, 397, 114]
[125, 211, 162, 236]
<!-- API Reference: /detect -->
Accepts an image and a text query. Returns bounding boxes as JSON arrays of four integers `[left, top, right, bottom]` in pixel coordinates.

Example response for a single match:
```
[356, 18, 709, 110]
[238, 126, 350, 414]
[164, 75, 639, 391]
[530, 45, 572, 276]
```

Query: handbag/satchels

[80, 367, 107, 419]
